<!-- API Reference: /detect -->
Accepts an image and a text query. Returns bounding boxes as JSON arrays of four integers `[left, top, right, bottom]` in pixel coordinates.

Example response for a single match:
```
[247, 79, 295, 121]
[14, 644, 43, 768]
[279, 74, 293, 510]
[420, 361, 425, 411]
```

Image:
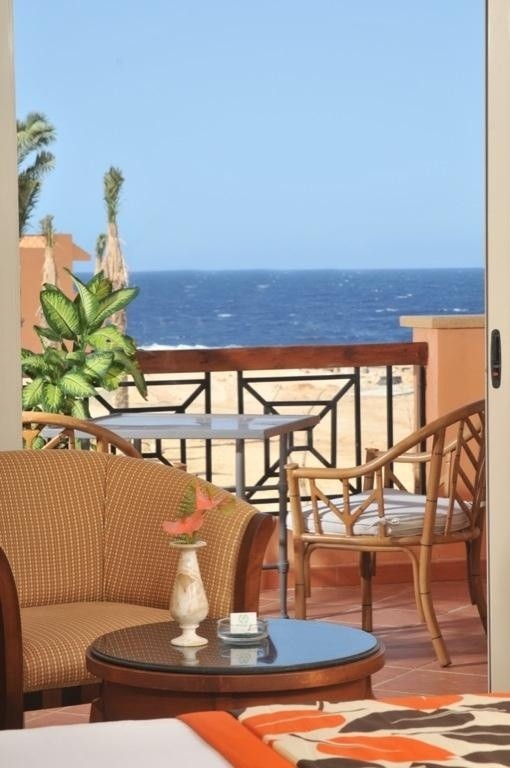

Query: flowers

[158, 480, 235, 543]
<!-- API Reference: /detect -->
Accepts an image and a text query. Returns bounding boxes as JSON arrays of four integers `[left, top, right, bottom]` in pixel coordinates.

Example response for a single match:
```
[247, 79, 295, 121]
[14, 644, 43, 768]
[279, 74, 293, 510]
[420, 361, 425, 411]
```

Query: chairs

[23, 409, 146, 461]
[283, 399, 486, 669]
[0, 448, 279, 730]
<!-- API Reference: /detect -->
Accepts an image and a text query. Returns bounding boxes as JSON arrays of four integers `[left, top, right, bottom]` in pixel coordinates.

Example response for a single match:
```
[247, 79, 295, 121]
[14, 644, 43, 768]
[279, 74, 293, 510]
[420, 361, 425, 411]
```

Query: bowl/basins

[216, 616, 270, 645]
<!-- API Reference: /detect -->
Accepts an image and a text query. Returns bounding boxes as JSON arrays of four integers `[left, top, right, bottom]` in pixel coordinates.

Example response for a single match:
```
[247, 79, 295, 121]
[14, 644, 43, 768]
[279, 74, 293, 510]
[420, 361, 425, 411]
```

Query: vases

[166, 542, 212, 648]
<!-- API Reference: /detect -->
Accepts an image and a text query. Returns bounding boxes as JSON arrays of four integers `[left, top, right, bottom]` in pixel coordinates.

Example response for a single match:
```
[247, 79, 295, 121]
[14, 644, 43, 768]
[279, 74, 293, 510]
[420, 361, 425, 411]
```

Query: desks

[95, 410, 321, 503]
